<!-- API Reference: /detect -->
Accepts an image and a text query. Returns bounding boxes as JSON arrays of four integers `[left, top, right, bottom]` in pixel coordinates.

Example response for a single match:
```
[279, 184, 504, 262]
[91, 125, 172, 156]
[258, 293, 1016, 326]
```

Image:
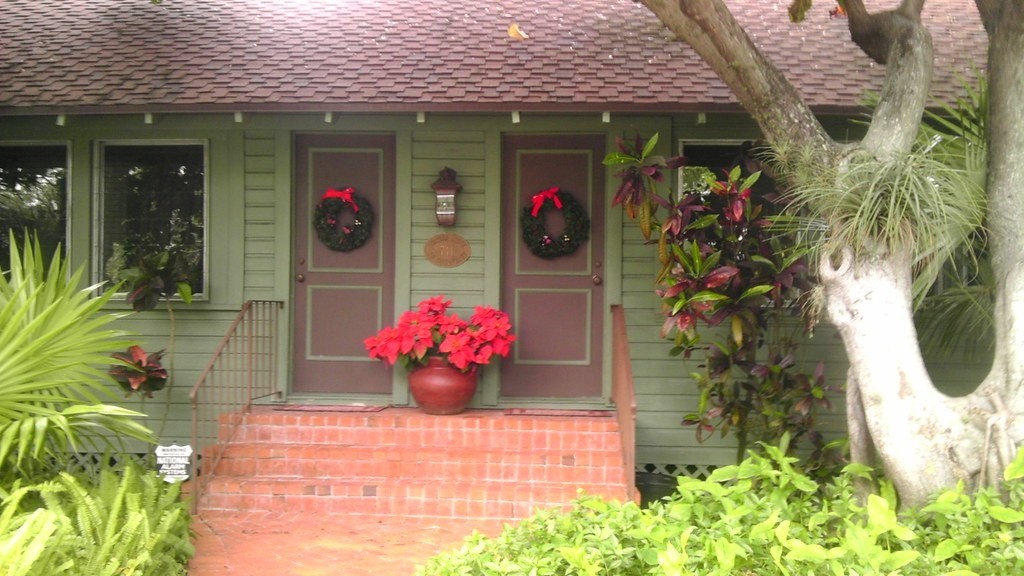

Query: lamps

[431, 166, 462, 226]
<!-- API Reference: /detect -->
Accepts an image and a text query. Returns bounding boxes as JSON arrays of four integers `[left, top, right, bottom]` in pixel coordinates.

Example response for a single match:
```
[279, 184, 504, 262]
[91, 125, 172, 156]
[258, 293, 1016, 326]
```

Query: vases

[408, 356, 477, 415]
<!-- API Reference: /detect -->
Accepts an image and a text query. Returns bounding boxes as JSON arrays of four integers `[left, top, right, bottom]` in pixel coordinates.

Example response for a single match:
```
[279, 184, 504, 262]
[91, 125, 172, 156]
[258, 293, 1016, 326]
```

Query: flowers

[362, 294, 519, 377]
